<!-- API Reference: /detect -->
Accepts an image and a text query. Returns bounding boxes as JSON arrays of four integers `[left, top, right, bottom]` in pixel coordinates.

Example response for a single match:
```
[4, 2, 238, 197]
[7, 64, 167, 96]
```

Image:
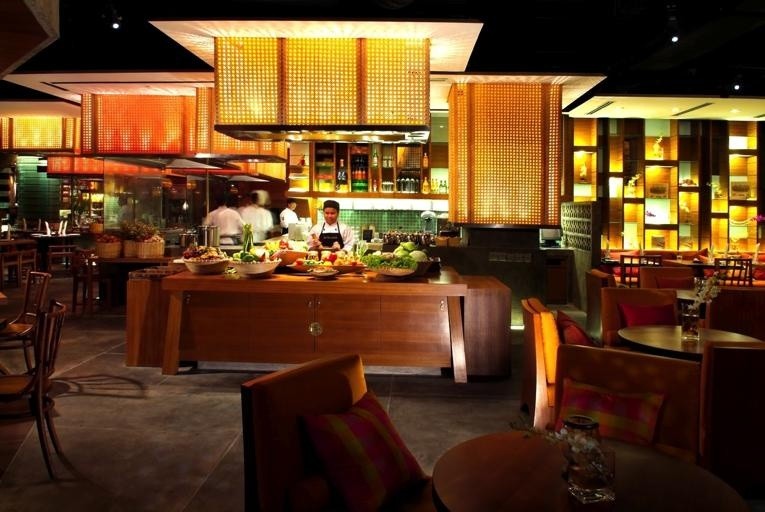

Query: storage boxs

[98, 242, 121, 261]
[125, 241, 165, 258]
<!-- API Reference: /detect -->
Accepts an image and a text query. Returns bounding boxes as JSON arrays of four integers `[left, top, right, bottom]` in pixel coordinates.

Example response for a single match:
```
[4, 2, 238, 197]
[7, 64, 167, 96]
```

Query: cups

[374, 233, 379, 239]
[579, 164, 589, 183]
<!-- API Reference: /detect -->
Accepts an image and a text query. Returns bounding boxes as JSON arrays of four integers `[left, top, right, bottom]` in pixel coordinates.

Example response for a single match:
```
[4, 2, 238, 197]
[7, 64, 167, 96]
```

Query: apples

[96, 233, 119, 243]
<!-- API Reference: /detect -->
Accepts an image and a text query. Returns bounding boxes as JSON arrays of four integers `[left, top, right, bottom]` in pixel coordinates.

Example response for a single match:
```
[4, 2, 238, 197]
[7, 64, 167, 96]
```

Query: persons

[306, 198, 355, 255]
[278, 199, 300, 235]
[201, 189, 274, 246]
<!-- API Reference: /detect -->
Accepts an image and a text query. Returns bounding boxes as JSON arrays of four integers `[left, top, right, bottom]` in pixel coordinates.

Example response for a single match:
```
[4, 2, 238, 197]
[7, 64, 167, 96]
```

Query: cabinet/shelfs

[566, 117, 763, 253]
[284, 141, 452, 200]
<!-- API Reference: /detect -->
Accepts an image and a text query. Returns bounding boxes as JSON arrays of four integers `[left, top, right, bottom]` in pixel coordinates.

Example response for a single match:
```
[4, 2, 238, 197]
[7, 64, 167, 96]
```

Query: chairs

[550, 343, 704, 468]
[241, 353, 443, 511]
[585, 249, 765, 339]
[698, 339, 765, 470]
[521, 297, 563, 434]
[600, 288, 679, 352]
[0, 214, 100, 316]
[1, 270, 52, 381]
[1, 299, 84, 485]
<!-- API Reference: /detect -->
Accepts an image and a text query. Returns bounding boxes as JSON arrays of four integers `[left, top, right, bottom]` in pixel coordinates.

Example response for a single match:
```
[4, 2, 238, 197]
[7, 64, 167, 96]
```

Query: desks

[617, 326, 765, 361]
[432, 430, 747, 511]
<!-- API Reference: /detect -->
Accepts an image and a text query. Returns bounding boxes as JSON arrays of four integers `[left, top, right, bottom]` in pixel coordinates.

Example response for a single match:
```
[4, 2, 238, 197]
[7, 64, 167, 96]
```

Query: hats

[323, 201, 339, 210]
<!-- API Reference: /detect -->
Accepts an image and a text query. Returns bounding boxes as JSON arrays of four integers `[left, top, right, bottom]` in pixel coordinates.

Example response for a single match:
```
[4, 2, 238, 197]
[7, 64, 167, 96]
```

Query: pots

[179, 224, 221, 248]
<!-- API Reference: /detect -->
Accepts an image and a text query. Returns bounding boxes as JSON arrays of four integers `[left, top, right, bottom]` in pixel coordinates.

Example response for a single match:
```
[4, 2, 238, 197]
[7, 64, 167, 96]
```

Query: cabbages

[394, 242, 427, 260]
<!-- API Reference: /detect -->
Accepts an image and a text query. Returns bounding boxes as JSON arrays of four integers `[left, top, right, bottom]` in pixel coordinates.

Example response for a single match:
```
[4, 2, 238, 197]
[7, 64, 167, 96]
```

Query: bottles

[384, 230, 432, 248]
[382, 155, 393, 169]
[336, 158, 346, 183]
[682, 177, 696, 185]
[372, 179, 378, 193]
[431, 178, 448, 194]
[731, 181, 752, 199]
[396, 173, 420, 194]
[422, 152, 428, 170]
[351, 155, 368, 192]
[372, 149, 378, 167]
[382, 181, 393, 192]
[422, 176, 429, 194]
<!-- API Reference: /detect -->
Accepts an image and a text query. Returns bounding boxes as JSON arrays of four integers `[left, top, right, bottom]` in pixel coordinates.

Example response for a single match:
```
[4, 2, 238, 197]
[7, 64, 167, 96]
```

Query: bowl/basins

[229, 259, 283, 278]
[404, 262, 434, 278]
[435, 236, 462, 248]
[182, 258, 230, 275]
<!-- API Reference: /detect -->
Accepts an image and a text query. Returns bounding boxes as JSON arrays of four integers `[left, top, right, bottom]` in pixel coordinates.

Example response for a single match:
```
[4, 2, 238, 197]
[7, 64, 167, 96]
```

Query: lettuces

[363, 256, 421, 269]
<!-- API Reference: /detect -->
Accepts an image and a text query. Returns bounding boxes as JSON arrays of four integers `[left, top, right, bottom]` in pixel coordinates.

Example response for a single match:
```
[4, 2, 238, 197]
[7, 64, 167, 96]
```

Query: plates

[308, 270, 340, 278]
[285, 263, 365, 275]
[373, 266, 415, 277]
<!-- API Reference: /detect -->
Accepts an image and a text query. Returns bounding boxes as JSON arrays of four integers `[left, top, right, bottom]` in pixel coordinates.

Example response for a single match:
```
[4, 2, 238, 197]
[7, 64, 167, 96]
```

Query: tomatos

[296, 258, 303, 265]
[328, 253, 336, 261]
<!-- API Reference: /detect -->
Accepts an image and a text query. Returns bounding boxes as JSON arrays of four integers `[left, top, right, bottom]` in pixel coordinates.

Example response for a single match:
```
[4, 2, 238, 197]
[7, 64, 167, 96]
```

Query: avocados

[233, 250, 259, 261]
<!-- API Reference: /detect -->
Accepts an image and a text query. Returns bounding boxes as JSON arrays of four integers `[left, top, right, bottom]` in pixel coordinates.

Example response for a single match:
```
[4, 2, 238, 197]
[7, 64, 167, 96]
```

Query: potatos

[333, 260, 343, 266]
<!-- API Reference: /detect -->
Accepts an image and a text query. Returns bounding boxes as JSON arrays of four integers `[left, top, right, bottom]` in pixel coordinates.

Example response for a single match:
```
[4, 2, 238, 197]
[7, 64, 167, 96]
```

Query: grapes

[184, 247, 230, 264]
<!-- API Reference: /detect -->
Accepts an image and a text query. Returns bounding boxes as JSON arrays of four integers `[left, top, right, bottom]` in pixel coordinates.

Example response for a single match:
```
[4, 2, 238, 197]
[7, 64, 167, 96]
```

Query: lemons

[324, 261, 332, 266]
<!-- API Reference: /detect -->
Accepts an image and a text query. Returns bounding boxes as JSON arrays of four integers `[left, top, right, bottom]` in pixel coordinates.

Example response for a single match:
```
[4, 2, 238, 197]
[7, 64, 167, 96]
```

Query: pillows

[617, 302, 677, 328]
[556, 377, 666, 448]
[303, 387, 432, 512]
[556, 308, 599, 349]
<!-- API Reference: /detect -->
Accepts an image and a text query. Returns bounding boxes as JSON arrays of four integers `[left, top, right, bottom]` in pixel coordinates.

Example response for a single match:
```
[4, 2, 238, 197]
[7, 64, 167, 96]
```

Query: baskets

[434, 236, 461, 247]
[95, 238, 165, 259]
